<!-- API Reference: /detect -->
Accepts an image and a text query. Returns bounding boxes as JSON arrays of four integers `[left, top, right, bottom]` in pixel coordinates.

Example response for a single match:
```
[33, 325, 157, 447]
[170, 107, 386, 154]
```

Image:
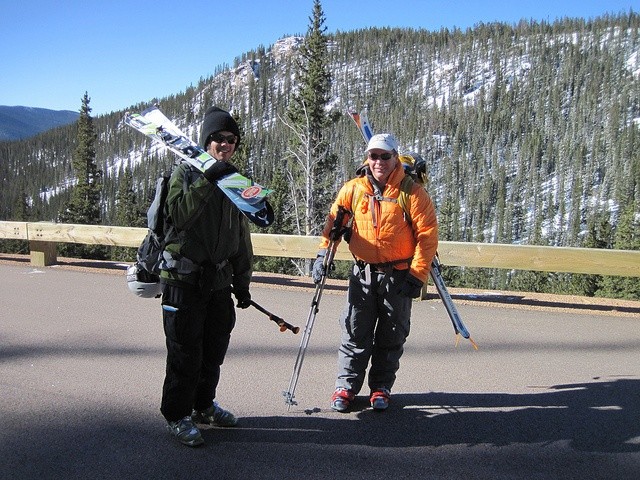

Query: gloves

[396, 272, 424, 298]
[237, 290, 251, 309]
[312, 249, 335, 284]
[203, 159, 239, 182]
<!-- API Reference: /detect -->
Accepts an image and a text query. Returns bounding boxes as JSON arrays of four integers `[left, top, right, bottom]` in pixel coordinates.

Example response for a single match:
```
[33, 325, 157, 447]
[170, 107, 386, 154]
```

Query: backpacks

[344, 152, 428, 245]
[136, 157, 197, 276]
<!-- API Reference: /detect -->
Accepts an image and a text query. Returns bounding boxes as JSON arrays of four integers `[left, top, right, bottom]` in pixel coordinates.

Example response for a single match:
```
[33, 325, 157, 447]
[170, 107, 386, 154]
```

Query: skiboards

[347, 107, 472, 340]
[123, 105, 274, 227]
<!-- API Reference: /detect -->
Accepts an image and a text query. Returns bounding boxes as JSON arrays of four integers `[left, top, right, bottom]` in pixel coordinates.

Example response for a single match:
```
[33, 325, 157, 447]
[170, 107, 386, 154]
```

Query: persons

[312, 133, 439, 413]
[129, 108, 254, 449]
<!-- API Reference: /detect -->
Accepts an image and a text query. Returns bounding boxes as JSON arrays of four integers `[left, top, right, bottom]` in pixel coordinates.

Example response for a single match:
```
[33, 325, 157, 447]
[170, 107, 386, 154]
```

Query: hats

[364, 134, 398, 155]
[201, 106, 240, 153]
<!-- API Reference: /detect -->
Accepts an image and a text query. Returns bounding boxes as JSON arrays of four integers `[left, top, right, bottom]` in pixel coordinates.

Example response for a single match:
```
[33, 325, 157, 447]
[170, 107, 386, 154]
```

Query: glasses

[367, 152, 395, 160]
[210, 133, 237, 144]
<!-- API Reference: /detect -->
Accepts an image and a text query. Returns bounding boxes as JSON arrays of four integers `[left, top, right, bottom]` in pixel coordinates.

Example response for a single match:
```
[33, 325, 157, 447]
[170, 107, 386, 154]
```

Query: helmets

[126, 262, 162, 298]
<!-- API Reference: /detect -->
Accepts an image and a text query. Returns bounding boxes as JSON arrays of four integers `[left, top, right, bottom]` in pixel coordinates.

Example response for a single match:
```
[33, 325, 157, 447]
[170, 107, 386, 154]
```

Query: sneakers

[167, 415, 204, 447]
[191, 402, 237, 427]
[331, 387, 355, 411]
[370, 385, 391, 410]
